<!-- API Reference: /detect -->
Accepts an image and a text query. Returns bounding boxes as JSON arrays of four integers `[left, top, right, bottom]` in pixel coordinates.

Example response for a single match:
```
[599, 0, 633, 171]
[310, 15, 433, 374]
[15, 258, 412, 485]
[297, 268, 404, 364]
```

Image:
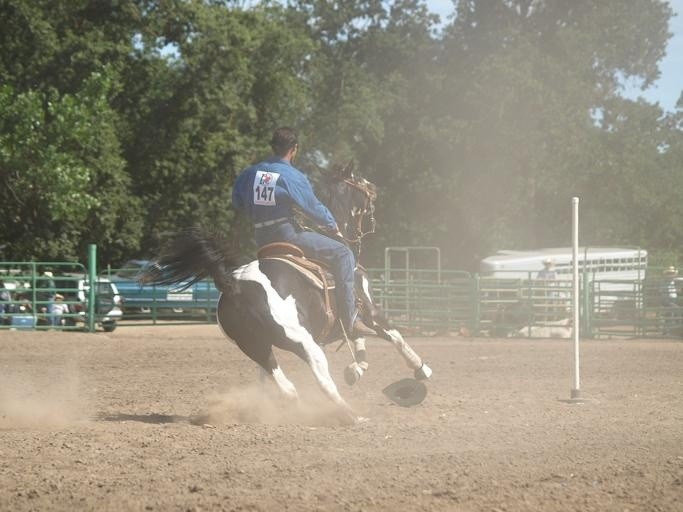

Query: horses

[132, 157, 433, 427]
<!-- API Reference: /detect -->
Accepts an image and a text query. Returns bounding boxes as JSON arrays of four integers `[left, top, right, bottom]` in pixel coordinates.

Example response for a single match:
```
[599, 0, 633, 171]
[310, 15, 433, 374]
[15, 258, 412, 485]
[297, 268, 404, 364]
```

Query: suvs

[9, 270, 122, 332]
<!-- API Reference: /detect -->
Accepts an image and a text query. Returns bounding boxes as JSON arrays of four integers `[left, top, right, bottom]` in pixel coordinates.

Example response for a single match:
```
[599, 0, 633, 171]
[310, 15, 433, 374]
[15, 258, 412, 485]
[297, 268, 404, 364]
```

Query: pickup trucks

[95, 259, 221, 321]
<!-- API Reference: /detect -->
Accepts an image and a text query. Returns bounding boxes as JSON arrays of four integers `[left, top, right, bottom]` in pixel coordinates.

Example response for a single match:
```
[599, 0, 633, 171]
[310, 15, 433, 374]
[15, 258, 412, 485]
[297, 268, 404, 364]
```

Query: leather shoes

[337, 321, 378, 336]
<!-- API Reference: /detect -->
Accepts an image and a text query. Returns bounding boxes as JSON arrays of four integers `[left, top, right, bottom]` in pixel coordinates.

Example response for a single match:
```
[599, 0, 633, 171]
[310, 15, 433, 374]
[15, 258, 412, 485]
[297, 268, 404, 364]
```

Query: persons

[537, 256, 558, 321]
[232, 126, 378, 338]
[661, 266, 683, 335]
[48, 294, 69, 325]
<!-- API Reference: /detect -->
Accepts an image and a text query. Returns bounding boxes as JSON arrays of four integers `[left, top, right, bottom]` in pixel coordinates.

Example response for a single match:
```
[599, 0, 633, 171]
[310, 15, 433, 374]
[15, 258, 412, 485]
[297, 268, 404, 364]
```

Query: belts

[254, 217, 289, 228]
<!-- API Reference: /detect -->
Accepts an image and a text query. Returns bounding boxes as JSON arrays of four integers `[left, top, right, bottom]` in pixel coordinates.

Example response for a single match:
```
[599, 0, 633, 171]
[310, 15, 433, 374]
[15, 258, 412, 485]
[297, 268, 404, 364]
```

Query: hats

[663, 267, 678, 275]
[54, 294, 64, 300]
[44, 271, 53, 278]
